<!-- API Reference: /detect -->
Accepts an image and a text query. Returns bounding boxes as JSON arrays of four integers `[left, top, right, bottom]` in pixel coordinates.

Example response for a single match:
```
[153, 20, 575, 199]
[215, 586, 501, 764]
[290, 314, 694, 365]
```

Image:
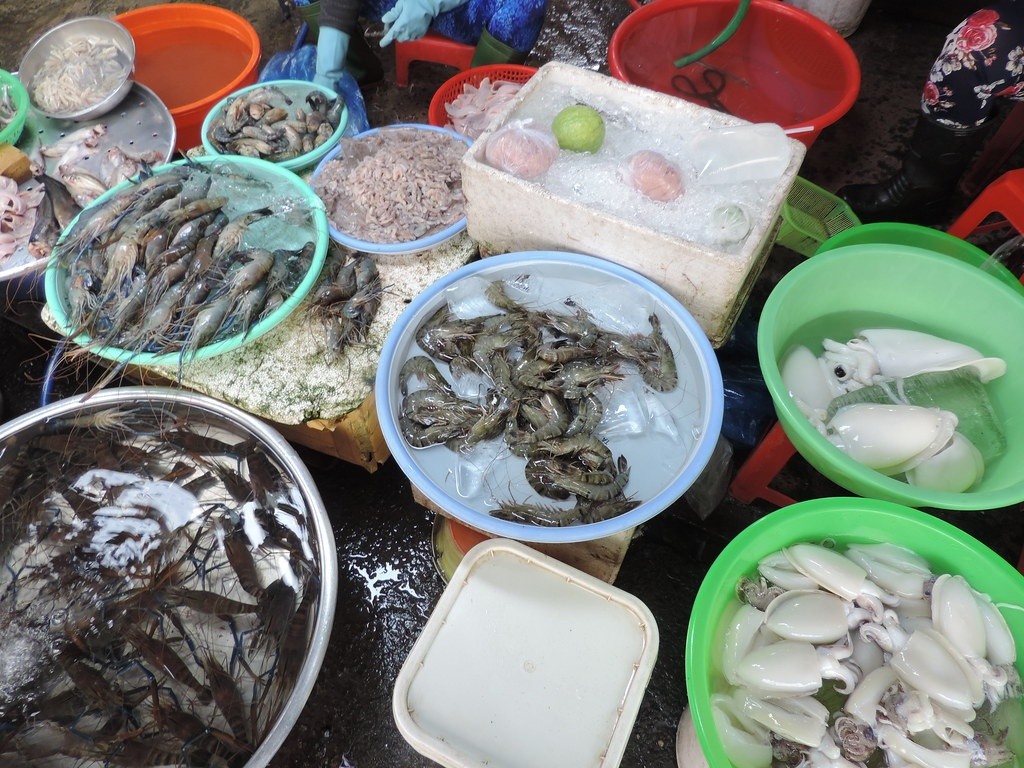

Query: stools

[949, 169, 1024, 286]
[394, 33, 477, 88]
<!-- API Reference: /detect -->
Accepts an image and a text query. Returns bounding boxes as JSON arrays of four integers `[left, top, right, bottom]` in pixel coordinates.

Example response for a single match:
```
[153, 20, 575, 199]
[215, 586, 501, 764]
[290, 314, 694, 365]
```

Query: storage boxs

[41, 228, 478, 473]
[463, 59, 807, 347]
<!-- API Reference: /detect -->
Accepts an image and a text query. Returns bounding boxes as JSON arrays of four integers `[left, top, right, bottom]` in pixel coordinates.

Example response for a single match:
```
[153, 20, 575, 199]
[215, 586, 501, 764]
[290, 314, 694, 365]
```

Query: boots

[834, 104, 1002, 224]
[470, 19, 528, 70]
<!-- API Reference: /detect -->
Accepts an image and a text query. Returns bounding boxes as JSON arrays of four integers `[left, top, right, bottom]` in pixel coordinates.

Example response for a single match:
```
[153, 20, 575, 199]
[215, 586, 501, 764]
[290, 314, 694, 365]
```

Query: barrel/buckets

[785, 0, 870, 39]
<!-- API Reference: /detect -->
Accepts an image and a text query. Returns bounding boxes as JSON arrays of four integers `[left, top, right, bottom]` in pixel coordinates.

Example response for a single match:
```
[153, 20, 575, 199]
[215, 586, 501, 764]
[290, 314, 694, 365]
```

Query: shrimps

[0, 149, 681, 768]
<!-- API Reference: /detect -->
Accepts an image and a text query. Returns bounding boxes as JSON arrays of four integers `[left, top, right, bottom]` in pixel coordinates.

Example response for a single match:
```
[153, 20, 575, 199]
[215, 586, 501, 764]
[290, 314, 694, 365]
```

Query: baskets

[0, 68, 30, 146]
[775, 176, 861, 257]
[428, 64, 538, 125]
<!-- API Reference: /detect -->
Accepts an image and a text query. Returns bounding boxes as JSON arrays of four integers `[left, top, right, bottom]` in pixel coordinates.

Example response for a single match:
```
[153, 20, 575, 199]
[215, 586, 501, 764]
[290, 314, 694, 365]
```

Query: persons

[312, 0, 548, 93]
[833, 0, 1024, 224]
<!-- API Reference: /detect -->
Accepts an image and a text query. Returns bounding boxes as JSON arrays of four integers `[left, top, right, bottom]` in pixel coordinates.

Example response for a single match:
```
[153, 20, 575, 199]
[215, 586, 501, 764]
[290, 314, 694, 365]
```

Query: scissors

[671, 66, 732, 114]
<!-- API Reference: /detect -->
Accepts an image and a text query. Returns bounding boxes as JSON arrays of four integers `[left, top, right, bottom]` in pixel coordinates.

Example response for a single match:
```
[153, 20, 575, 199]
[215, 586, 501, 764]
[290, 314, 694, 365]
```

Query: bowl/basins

[682, 496, 1024, 768]
[307, 122, 476, 254]
[375, 251, 725, 544]
[112, 4, 261, 152]
[19, 16, 136, 121]
[608, 0, 860, 154]
[201, 78, 347, 175]
[45, 155, 326, 365]
[808, 222, 1024, 292]
[0, 384, 338, 768]
[757, 242, 1024, 511]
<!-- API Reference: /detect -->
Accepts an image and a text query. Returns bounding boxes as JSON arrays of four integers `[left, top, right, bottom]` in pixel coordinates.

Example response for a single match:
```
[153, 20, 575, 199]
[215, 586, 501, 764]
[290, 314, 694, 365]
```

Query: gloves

[312, 27, 350, 92]
[380, 0, 467, 48]
